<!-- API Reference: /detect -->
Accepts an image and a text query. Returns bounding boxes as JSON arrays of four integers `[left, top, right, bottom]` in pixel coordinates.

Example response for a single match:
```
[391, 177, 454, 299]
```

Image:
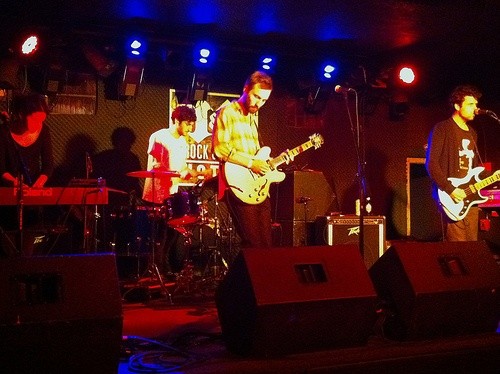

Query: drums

[104, 207, 150, 271]
[167, 184, 202, 226]
[130, 204, 170, 241]
[159, 220, 224, 279]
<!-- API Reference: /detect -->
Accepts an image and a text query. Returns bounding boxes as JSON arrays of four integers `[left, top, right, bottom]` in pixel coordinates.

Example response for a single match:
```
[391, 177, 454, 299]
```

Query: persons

[426, 86, 500, 240]
[0, 95, 54, 230]
[213, 71, 294, 248]
[134, 106, 197, 273]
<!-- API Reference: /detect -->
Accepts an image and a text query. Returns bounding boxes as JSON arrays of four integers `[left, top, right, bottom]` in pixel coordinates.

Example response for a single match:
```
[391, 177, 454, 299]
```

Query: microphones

[335, 84, 355, 93]
[474, 108, 494, 115]
[86, 152, 93, 173]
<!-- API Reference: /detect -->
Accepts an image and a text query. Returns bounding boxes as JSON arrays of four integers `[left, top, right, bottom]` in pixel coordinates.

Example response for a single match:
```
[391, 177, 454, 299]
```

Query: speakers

[367, 240, 500, 340]
[0, 252, 124, 374]
[214, 246, 377, 357]
[270, 171, 386, 259]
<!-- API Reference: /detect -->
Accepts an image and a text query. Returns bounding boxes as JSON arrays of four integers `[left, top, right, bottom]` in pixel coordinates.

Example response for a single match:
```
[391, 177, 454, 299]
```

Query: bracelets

[248, 160, 253, 168]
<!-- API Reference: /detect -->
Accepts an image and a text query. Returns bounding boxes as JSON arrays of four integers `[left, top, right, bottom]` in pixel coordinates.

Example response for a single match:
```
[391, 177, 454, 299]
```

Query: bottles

[355, 196, 360, 216]
[364, 197, 373, 215]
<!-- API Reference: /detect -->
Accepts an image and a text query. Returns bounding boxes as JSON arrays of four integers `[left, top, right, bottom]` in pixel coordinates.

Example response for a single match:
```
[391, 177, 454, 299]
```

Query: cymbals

[127, 171, 180, 179]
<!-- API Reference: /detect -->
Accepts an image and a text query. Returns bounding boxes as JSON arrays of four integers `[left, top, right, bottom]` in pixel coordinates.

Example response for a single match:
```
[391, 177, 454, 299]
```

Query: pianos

[0, 186, 109, 255]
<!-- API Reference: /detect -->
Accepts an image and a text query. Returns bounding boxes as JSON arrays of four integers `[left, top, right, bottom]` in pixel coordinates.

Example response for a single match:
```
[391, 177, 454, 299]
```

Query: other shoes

[165, 275, 176, 287]
[192, 275, 208, 284]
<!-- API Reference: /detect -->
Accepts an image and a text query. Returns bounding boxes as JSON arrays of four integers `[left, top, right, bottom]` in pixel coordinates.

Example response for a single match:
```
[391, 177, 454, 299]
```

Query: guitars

[222, 132, 325, 206]
[436, 166, 500, 222]
